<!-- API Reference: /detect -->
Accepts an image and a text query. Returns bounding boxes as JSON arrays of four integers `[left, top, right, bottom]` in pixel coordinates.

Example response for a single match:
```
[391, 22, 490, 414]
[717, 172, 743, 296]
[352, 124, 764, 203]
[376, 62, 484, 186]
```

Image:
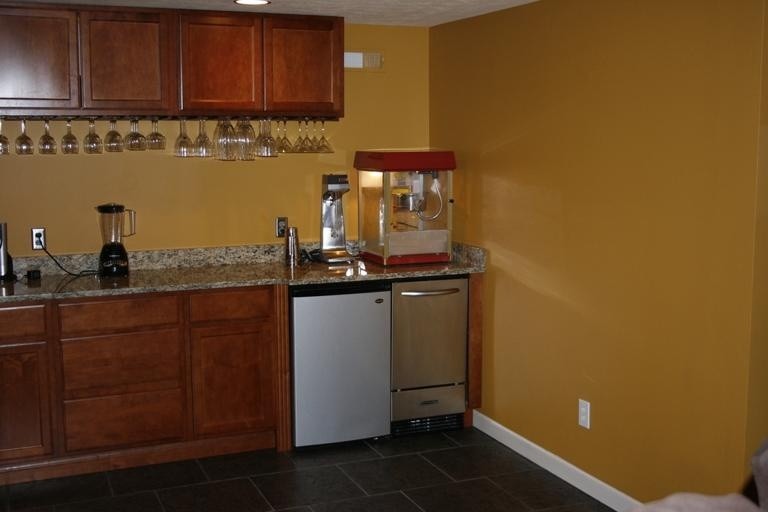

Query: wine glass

[301, 117, 313, 152]
[15, 118, 34, 154]
[293, 120, 303, 153]
[147, 117, 165, 149]
[263, 118, 278, 156]
[218, 116, 237, 161]
[212, 118, 223, 159]
[238, 117, 256, 161]
[256, 120, 261, 156]
[0, 116, 9, 155]
[62, 120, 79, 154]
[234, 118, 242, 132]
[39, 120, 57, 154]
[104, 120, 124, 152]
[257, 120, 266, 157]
[276, 119, 281, 144]
[174, 117, 194, 157]
[125, 117, 146, 150]
[311, 117, 320, 153]
[236, 117, 244, 133]
[278, 118, 292, 153]
[318, 118, 335, 152]
[84, 120, 103, 154]
[194, 118, 211, 156]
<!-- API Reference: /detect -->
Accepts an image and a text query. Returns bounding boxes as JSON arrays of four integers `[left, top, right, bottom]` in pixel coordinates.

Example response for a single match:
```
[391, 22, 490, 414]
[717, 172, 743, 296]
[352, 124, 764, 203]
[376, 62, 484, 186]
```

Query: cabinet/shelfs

[0, 1, 176, 121]
[180, 0, 350, 119]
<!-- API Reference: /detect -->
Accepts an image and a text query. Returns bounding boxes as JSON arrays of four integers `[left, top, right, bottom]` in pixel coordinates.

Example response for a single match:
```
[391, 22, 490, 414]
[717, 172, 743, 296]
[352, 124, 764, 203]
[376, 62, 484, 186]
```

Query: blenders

[94, 203, 135, 274]
[323, 263, 354, 277]
[310, 174, 353, 263]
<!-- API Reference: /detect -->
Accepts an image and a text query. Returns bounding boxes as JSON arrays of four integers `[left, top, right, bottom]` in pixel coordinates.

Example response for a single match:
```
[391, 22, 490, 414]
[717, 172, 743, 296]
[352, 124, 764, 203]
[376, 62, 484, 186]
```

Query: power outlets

[30, 224, 48, 256]
[274, 214, 291, 246]
[578, 399, 591, 429]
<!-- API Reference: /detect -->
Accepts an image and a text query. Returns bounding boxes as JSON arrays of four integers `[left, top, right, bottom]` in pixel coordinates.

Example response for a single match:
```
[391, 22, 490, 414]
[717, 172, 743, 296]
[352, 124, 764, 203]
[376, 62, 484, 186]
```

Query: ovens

[390, 276, 470, 435]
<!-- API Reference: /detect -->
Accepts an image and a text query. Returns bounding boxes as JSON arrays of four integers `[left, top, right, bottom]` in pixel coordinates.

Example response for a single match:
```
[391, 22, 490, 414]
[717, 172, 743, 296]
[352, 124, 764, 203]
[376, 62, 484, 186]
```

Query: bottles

[287, 227, 299, 265]
